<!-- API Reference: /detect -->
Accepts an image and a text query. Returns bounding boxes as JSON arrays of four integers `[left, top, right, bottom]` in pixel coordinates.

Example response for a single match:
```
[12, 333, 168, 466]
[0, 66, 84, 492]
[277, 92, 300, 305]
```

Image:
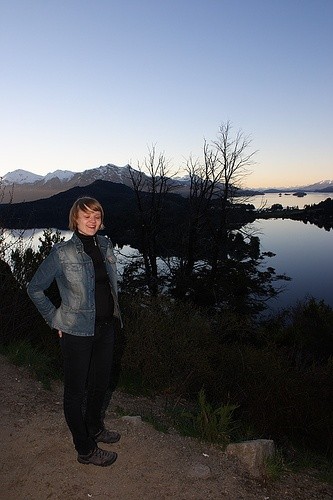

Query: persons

[26, 197, 123, 468]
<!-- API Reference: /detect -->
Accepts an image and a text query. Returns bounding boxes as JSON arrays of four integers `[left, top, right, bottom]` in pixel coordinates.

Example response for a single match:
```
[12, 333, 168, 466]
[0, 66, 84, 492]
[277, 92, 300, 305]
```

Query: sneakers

[78, 447, 117, 467]
[96, 429, 121, 443]
